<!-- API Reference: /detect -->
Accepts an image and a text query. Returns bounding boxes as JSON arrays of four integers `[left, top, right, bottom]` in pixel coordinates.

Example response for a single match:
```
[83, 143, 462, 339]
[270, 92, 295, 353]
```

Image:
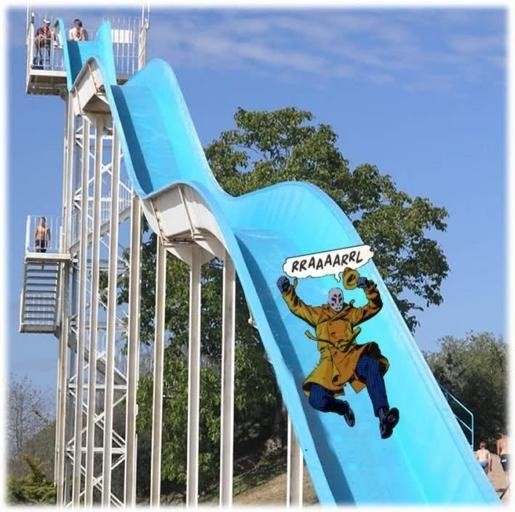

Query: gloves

[357, 277, 368, 288]
[277, 276, 290, 292]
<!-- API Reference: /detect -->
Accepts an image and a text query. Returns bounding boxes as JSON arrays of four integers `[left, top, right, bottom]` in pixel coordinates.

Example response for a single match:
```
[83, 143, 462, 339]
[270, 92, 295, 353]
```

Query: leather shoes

[341, 400, 355, 427]
[378, 405, 399, 438]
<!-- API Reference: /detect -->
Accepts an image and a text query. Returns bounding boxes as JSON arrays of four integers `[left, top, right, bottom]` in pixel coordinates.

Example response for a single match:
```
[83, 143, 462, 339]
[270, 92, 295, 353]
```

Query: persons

[68, 17, 88, 41]
[497, 430, 510, 471]
[36, 19, 55, 69]
[35, 217, 51, 253]
[277, 267, 401, 439]
[476, 441, 491, 473]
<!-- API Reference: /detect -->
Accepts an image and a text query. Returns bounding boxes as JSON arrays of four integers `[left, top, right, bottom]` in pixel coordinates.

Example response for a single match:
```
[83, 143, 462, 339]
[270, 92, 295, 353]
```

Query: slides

[52, 17, 502, 507]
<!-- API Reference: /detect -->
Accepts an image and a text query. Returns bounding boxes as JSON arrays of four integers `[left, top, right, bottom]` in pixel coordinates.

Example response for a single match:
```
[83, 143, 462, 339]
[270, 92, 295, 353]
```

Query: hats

[343, 266, 360, 290]
